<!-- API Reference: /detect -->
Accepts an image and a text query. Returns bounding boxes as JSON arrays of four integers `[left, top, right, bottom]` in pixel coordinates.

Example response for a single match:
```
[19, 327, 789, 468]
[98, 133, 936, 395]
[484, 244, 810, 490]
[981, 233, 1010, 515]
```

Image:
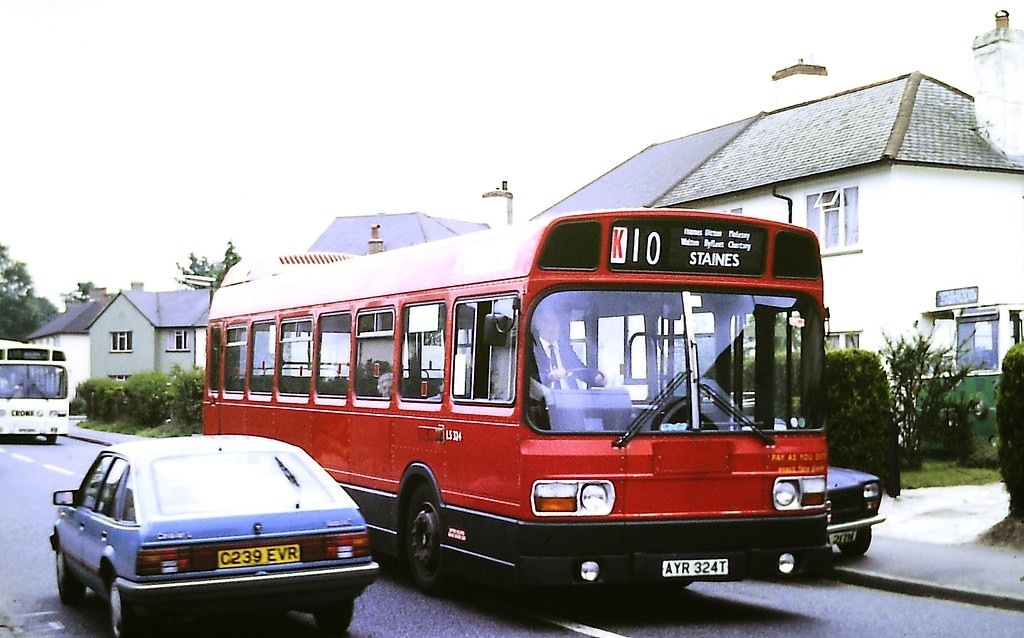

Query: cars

[50, 433, 381, 638]
[720, 415, 886, 567]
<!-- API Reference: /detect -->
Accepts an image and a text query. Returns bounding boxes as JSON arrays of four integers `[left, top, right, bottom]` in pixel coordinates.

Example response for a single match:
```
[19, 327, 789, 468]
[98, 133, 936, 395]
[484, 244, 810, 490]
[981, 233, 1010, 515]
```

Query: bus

[0, 339, 70, 447]
[202, 207, 836, 613]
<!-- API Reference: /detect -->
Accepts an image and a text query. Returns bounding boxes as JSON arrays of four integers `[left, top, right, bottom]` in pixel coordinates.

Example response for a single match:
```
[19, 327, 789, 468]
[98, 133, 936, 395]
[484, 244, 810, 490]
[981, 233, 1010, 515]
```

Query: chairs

[232, 373, 441, 398]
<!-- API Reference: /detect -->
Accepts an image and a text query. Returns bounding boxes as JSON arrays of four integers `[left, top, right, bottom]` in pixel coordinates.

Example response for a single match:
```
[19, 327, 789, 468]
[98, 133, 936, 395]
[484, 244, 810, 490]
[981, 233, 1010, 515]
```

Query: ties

[548, 345, 561, 391]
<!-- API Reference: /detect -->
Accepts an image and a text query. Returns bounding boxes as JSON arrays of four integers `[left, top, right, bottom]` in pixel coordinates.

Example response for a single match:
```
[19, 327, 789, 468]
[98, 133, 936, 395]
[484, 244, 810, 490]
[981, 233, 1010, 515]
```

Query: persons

[532, 305, 606, 390]
[379, 374, 405, 397]
